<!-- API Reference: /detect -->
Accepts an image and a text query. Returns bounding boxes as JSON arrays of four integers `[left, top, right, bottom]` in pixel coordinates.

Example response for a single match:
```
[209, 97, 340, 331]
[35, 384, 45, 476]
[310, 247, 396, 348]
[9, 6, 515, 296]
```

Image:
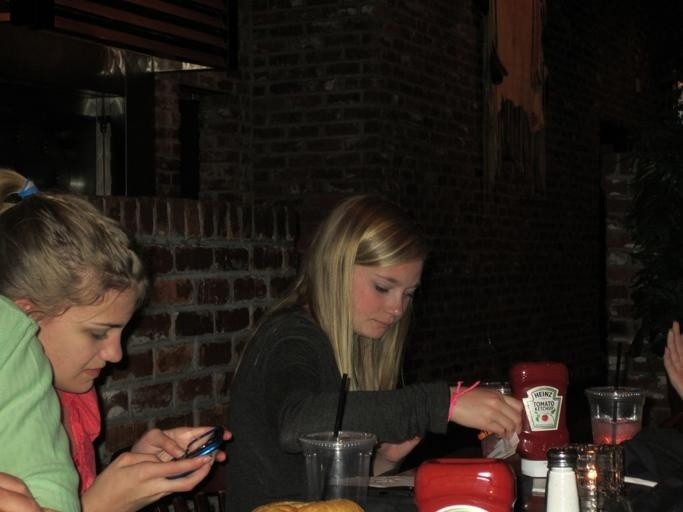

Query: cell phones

[167, 425, 224, 480]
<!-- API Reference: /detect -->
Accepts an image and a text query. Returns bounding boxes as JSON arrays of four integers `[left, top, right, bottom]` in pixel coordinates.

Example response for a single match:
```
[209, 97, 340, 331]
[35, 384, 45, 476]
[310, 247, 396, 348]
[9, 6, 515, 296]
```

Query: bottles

[509, 359, 575, 480]
[542, 446, 581, 512]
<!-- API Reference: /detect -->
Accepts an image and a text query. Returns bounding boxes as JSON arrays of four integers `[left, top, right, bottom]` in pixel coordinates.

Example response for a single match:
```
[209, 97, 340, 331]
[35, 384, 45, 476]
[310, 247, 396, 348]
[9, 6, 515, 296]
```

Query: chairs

[138, 464, 228, 512]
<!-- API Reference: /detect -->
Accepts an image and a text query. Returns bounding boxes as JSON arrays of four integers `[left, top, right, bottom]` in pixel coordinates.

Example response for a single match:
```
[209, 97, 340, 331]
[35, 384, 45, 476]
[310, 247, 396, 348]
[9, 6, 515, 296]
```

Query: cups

[475, 380, 514, 457]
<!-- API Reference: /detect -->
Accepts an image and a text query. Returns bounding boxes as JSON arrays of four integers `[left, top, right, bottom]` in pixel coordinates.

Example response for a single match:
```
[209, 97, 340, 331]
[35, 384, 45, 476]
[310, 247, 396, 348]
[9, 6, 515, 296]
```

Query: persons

[616, 319, 683, 512]
[223, 193, 524, 512]
[1, 295, 83, 511]
[0, 167, 233, 512]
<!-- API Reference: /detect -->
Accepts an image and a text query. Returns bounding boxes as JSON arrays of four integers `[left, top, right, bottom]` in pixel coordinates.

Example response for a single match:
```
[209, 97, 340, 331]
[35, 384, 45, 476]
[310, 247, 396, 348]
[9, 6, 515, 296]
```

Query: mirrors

[0, 21, 250, 202]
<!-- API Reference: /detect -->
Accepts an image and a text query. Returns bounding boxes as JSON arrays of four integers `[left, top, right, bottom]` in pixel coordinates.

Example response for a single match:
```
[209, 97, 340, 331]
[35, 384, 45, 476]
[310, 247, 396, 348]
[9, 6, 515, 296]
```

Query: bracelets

[446, 379, 482, 426]
